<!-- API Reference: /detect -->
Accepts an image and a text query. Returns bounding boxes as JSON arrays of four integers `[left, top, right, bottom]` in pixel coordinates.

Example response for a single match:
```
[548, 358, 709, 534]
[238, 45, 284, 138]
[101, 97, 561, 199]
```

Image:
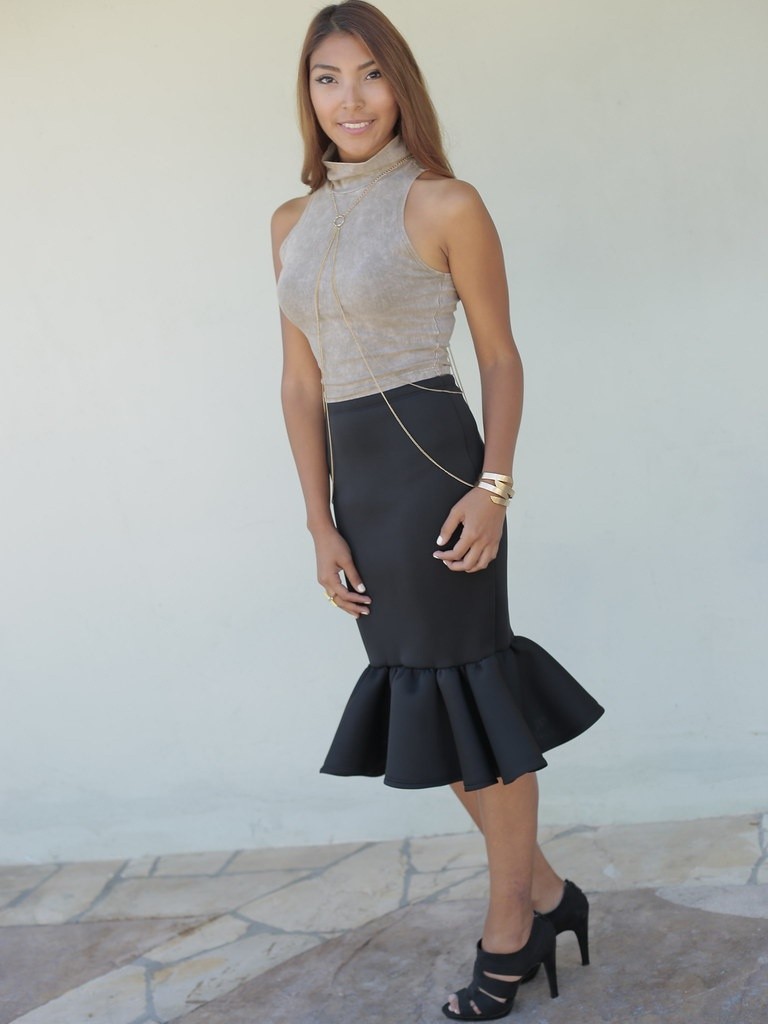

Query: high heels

[520, 879, 590, 985]
[441, 911, 558, 1021]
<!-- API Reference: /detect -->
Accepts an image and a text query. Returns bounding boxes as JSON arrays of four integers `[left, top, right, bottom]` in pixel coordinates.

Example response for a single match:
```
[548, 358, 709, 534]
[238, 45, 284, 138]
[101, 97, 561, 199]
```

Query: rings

[322, 592, 337, 610]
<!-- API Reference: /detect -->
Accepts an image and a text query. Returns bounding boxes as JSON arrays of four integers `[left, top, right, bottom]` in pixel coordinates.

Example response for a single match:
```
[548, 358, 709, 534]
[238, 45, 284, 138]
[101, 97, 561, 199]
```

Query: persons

[268, 1, 603, 1021]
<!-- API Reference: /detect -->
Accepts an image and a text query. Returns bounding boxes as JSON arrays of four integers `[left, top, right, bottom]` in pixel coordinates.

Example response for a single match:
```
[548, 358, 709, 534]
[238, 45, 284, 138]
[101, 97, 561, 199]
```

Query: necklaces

[311, 149, 475, 500]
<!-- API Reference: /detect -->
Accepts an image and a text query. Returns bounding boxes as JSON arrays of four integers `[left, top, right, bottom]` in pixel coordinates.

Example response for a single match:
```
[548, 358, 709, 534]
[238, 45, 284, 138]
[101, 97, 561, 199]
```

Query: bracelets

[476, 472, 515, 509]
